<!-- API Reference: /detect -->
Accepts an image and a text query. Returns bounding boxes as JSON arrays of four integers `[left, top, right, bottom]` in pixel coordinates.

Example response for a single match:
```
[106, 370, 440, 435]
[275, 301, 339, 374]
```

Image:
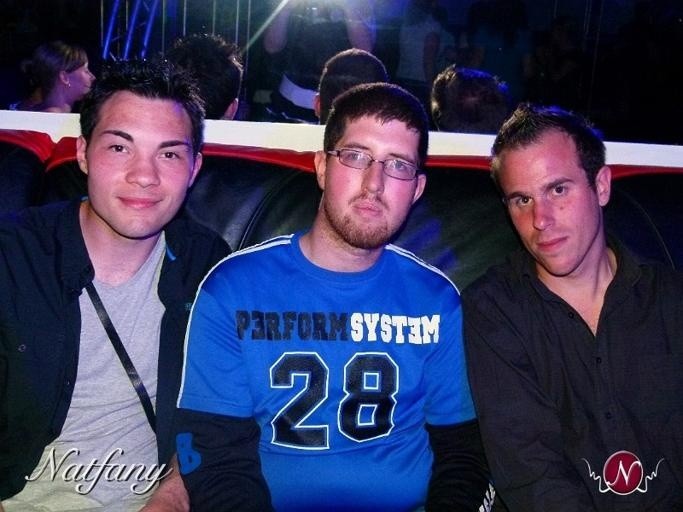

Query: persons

[2, 53, 233, 512]
[18, 39, 98, 113]
[396, 0, 440, 82]
[259, 1, 375, 122]
[173, 80, 501, 512]
[458, 103, 682, 512]
[424, 62, 511, 132]
[12, 72, 44, 110]
[310, 49, 390, 125]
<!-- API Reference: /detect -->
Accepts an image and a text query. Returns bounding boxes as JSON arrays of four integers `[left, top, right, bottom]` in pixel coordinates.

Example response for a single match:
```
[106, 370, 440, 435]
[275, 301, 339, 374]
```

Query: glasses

[326, 148, 421, 181]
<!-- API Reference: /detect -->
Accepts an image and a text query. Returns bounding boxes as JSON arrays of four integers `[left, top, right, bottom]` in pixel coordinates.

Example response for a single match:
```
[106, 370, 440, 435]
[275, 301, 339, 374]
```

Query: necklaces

[161, 33, 245, 119]
